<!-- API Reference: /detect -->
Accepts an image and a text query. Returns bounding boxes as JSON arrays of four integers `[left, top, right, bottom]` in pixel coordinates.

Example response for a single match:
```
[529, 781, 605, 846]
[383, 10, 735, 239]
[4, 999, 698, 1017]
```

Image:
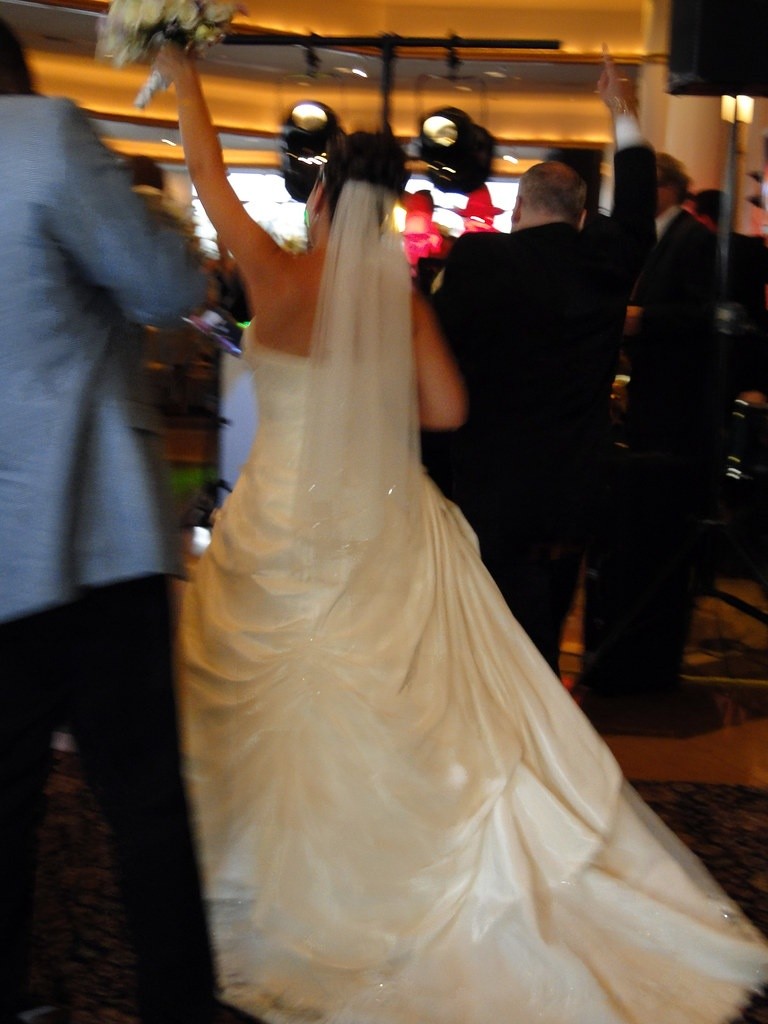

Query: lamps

[420, 104, 496, 193]
[279, 100, 339, 202]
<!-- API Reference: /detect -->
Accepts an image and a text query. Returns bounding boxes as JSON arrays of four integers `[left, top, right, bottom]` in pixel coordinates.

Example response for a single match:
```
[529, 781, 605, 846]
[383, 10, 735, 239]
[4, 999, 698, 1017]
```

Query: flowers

[95, 0, 250, 108]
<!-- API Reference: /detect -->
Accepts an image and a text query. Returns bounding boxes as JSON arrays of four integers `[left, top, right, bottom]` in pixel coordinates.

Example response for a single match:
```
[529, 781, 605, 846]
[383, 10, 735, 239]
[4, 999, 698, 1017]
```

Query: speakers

[665, 0, 768, 99]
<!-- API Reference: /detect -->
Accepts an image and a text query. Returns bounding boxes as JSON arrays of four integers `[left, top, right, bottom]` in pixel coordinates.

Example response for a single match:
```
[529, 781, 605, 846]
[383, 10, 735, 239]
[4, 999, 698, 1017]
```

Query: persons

[410, 190, 456, 264]
[420, 52, 731, 703]
[0, 23, 263, 1024]
[148, 26, 463, 1024]
